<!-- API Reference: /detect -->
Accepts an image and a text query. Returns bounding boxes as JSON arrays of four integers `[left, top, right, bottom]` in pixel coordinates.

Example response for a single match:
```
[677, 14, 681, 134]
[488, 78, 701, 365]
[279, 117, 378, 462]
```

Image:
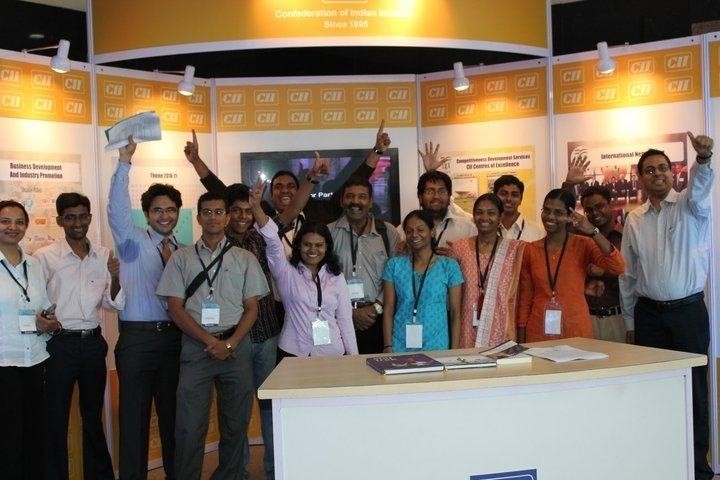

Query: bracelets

[383, 346, 392, 350]
[697, 151, 713, 158]
[301, 174, 321, 186]
[46, 322, 62, 336]
[372, 147, 384, 157]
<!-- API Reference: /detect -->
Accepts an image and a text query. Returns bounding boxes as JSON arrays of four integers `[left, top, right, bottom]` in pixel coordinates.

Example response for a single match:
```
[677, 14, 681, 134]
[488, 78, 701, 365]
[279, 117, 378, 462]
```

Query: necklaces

[547, 243, 563, 263]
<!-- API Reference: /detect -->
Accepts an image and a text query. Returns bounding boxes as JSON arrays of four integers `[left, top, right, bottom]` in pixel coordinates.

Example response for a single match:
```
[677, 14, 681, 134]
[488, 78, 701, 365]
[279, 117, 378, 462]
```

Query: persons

[183, 119, 391, 332]
[382, 210, 465, 354]
[517, 189, 626, 343]
[248, 177, 359, 366]
[618, 131, 715, 480]
[417, 142, 533, 243]
[577, 164, 688, 198]
[224, 151, 329, 479]
[326, 178, 401, 353]
[447, 193, 531, 349]
[32, 193, 125, 480]
[155, 191, 270, 480]
[395, 170, 479, 257]
[561, 154, 626, 344]
[0, 199, 63, 480]
[107, 135, 191, 480]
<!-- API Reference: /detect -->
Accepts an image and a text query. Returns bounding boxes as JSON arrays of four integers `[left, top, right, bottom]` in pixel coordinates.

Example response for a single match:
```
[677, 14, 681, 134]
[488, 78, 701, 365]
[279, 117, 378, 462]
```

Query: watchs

[224, 339, 233, 353]
[374, 303, 384, 315]
[589, 227, 600, 238]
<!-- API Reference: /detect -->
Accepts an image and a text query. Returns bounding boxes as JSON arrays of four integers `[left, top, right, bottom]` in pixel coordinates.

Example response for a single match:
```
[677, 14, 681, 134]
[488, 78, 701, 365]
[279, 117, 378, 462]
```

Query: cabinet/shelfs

[256, 338, 708, 480]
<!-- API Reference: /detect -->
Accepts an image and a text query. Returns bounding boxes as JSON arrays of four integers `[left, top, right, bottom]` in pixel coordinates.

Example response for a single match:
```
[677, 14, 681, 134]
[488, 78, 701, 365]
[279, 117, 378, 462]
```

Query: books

[366, 354, 445, 374]
[424, 352, 496, 369]
[478, 341, 533, 364]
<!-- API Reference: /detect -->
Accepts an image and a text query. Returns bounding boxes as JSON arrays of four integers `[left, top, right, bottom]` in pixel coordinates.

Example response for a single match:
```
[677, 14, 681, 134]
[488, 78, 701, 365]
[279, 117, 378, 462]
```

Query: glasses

[641, 165, 670, 175]
[424, 189, 448, 195]
[150, 207, 177, 214]
[199, 209, 226, 217]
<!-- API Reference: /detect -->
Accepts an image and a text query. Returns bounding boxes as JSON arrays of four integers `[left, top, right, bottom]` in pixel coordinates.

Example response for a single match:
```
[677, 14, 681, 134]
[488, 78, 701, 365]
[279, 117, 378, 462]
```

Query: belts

[210, 327, 237, 341]
[352, 301, 366, 309]
[123, 322, 174, 333]
[589, 306, 621, 317]
[654, 294, 703, 311]
[62, 326, 101, 339]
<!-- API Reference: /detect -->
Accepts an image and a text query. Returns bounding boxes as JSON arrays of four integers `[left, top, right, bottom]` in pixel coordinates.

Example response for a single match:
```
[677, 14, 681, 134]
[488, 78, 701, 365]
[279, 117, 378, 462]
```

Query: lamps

[596, 41, 629, 74]
[22, 40, 71, 74]
[452, 60, 484, 91]
[155, 65, 195, 96]
